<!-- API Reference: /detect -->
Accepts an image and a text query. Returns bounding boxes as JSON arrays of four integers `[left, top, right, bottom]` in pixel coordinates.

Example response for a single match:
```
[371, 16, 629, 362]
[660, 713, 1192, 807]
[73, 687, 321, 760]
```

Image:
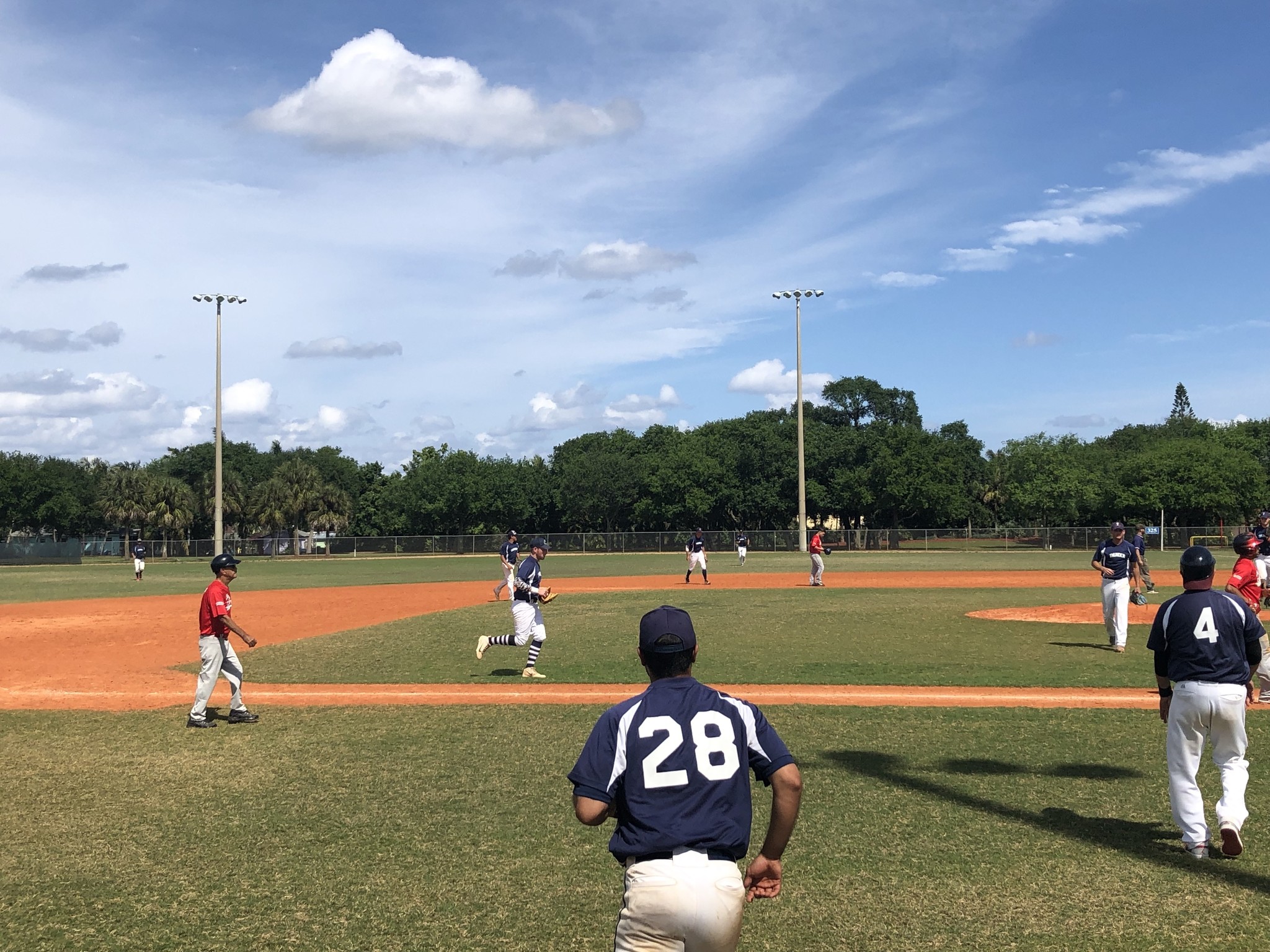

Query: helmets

[1232, 532, 1264, 557]
[211, 554, 242, 572]
[1179, 546, 1216, 580]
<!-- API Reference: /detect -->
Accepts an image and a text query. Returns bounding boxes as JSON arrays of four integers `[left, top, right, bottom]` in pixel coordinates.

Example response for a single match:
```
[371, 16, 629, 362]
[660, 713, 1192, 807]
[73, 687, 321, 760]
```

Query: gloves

[1262, 588, 1270, 598]
[1248, 598, 1261, 614]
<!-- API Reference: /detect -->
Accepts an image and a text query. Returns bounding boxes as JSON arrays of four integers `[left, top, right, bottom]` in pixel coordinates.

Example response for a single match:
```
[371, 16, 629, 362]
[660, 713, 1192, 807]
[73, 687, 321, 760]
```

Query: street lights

[194, 294, 247, 559]
[773, 289, 824, 552]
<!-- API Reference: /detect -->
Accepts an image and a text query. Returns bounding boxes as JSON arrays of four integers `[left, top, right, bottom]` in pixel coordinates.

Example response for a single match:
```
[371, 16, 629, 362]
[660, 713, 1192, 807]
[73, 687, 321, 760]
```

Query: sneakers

[685, 577, 711, 585]
[475, 634, 492, 660]
[1109, 636, 1125, 653]
[186, 709, 259, 728]
[810, 582, 825, 587]
[493, 588, 499, 600]
[1184, 820, 1243, 859]
[522, 667, 546, 678]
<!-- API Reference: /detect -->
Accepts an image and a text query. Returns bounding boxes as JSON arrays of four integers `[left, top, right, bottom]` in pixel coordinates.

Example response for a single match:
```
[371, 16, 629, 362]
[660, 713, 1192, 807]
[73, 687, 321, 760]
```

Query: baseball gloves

[1129, 589, 1148, 605]
[823, 547, 831, 555]
[704, 557, 708, 563]
[515, 559, 522, 567]
[538, 586, 559, 605]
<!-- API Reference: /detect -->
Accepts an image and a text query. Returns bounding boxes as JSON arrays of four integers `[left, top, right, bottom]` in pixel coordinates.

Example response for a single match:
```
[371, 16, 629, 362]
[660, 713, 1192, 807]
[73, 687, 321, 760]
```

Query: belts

[200, 634, 228, 640]
[635, 849, 736, 863]
[1102, 575, 1127, 580]
[514, 598, 537, 603]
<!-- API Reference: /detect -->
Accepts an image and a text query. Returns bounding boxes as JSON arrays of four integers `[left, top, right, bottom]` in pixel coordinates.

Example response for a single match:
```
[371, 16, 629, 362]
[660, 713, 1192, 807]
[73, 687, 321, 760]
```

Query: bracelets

[1158, 683, 1173, 697]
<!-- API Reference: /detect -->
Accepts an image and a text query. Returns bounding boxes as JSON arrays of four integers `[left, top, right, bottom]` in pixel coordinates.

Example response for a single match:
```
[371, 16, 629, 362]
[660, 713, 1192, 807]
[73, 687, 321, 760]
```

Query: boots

[1146, 583, 1160, 594]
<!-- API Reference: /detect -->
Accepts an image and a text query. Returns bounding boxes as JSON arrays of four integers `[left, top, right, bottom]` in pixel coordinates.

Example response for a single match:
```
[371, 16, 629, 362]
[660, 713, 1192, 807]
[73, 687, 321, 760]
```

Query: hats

[1181, 573, 1213, 590]
[137, 538, 142, 541]
[639, 605, 696, 653]
[1111, 522, 1147, 530]
[530, 537, 552, 550]
[739, 529, 743, 532]
[508, 530, 518, 539]
[1259, 512, 1268, 520]
[696, 527, 702, 532]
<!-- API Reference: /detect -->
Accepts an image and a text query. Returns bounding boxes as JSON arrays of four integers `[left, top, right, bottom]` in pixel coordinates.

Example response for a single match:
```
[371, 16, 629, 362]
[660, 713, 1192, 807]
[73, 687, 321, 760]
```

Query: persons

[475, 537, 552, 678]
[685, 527, 711, 585]
[566, 605, 805, 952]
[493, 530, 523, 601]
[1091, 522, 1142, 653]
[131, 538, 146, 581]
[735, 529, 750, 566]
[809, 527, 831, 587]
[1246, 511, 1270, 607]
[1146, 546, 1266, 858]
[1128, 523, 1159, 594]
[186, 553, 259, 727]
[1224, 532, 1270, 703]
